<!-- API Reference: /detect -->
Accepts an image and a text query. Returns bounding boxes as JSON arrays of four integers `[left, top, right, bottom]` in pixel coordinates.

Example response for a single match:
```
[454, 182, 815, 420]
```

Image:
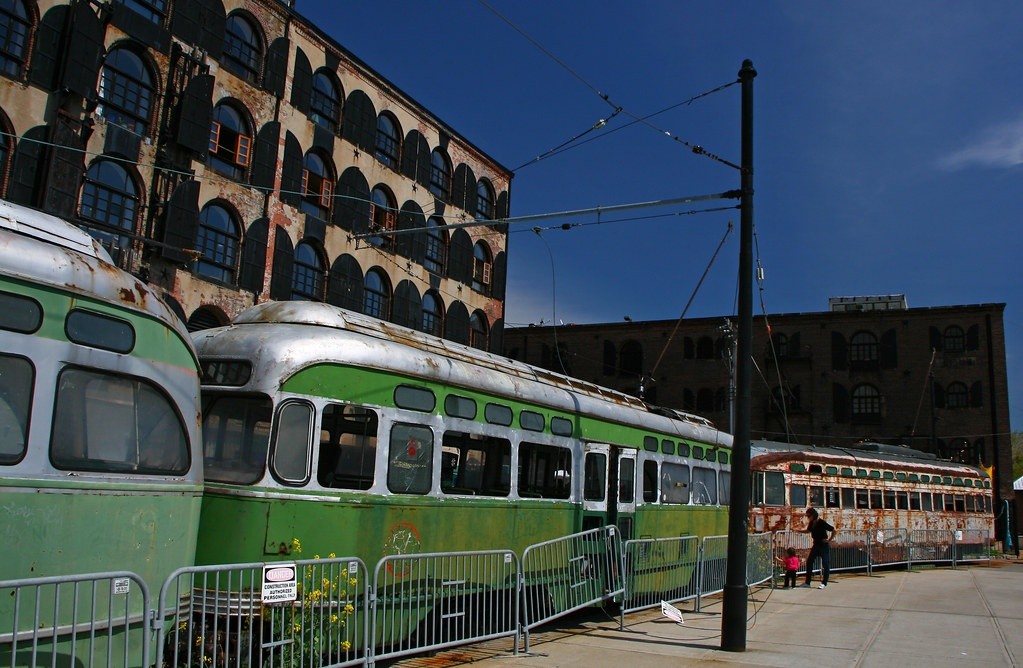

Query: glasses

[807, 514, 814, 518]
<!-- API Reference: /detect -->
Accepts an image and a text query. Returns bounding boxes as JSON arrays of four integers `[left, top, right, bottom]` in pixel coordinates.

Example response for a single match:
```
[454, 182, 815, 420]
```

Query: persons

[774, 546, 800, 589]
[790, 507, 837, 589]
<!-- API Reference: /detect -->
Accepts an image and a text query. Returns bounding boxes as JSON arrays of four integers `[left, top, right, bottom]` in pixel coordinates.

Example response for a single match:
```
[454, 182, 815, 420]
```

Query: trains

[1, 198, 994, 668]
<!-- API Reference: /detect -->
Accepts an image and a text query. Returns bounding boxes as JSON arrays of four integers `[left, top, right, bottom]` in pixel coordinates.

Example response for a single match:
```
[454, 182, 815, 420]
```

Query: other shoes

[800, 584, 810, 588]
[816, 583, 829, 589]
